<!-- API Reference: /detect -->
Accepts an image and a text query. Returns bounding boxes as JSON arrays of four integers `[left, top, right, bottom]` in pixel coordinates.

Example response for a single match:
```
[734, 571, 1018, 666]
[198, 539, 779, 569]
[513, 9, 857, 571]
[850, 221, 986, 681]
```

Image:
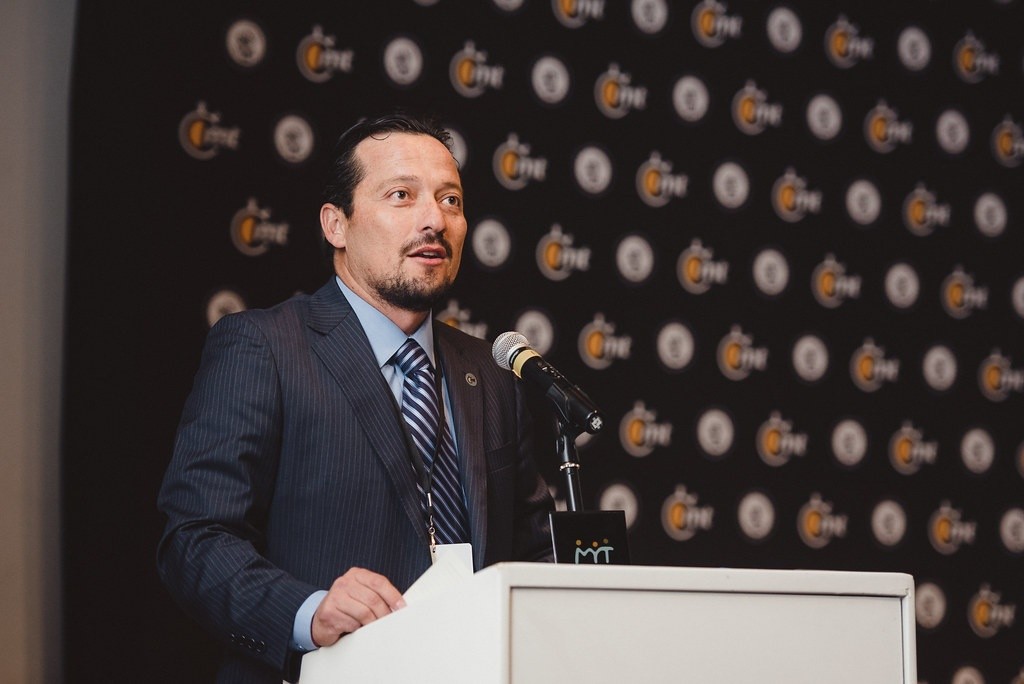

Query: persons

[159, 111, 559, 684]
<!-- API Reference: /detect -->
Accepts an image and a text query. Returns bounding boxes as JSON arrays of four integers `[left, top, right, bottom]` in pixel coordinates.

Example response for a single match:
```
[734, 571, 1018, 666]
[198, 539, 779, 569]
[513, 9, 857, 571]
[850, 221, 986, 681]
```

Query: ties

[393, 338, 470, 545]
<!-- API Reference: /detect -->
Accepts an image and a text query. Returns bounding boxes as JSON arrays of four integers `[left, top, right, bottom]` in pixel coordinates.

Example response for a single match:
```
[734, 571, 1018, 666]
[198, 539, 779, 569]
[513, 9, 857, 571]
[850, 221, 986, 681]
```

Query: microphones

[492, 331, 606, 435]
